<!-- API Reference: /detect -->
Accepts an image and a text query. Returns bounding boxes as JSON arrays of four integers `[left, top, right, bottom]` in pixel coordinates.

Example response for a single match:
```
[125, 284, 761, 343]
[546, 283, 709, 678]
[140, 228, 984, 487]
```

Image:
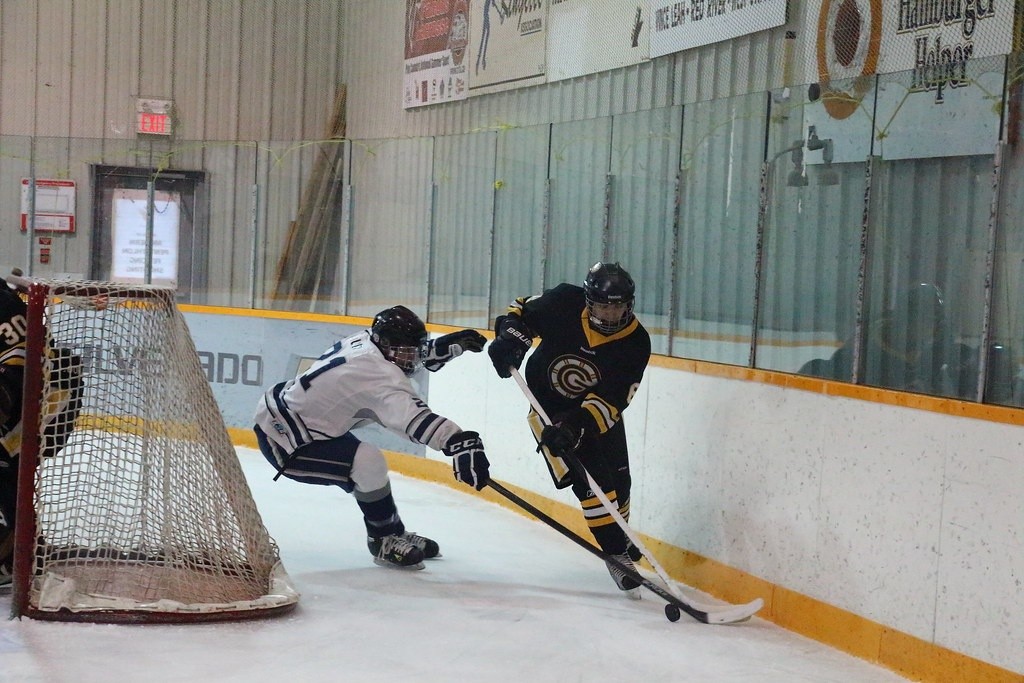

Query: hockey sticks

[486, 477, 766, 625]
[508, 361, 753, 624]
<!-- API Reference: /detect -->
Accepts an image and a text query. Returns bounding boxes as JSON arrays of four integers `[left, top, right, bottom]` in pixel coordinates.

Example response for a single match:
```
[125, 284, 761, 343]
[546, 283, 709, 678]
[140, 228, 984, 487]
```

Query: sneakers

[1, 529, 48, 594]
[620, 534, 643, 566]
[604, 548, 643, 601]
[393, 520, 444, 558]
[361, 515, 426, 571]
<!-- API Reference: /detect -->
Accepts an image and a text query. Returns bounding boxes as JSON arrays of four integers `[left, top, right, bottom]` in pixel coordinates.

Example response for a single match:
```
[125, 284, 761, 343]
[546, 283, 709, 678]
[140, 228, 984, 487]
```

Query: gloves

[535, 415, 586, 457]
[487, 324, 534, 378]
[442, 431, 490, 492]
[419, 328, 488, 372]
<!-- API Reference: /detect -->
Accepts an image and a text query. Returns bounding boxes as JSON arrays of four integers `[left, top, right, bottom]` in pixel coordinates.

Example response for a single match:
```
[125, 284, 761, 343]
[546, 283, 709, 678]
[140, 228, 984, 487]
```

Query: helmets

[584, 261, 635, 336]
[369, 304, 426, 375]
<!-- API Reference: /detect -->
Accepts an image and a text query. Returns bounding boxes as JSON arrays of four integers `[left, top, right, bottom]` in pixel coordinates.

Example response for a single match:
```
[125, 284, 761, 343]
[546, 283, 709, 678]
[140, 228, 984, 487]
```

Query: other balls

[663, 603, 682, 622]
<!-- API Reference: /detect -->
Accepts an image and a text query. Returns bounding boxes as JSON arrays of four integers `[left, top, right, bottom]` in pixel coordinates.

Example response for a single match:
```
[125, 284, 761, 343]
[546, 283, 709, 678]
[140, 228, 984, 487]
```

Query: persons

[253, 303, 491, 572]
[488, 262, 653, 599]
[0, 267, 88, 592]
[798, 281, 1024, 408]
